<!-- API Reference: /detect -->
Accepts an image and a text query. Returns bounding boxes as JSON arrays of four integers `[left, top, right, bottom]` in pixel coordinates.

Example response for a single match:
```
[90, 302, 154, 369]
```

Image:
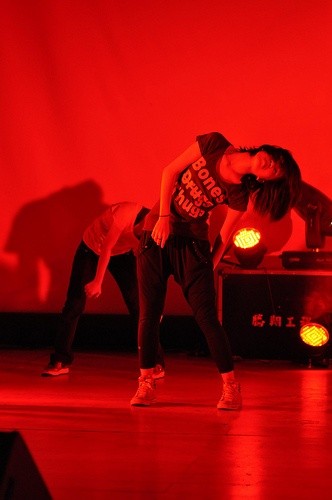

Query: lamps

[231, 228, 267, 269]
[298, 321, 331, 357]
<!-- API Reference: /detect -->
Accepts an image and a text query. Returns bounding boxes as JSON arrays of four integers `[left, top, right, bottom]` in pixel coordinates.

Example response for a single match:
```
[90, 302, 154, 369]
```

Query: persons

[40, 203, 159, 378]
[130, 132, 303, 410]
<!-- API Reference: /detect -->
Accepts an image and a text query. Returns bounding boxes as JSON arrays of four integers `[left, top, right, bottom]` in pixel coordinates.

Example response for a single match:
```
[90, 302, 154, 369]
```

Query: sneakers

[131, 374, 158, 405]
[217, 377, 241, 410]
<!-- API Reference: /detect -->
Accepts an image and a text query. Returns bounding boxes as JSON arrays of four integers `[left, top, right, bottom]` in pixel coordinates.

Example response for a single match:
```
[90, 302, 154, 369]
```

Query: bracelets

[159, 214, 170, 218]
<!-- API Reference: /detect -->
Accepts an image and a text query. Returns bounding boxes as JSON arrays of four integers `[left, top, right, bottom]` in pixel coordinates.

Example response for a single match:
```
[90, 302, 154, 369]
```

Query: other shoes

[41, 361, 69, 376]
[152, 363, 165, 379]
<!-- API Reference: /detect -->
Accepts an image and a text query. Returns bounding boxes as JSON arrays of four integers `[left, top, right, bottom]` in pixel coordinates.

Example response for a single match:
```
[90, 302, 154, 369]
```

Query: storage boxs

[216, 257, 332, 361]
